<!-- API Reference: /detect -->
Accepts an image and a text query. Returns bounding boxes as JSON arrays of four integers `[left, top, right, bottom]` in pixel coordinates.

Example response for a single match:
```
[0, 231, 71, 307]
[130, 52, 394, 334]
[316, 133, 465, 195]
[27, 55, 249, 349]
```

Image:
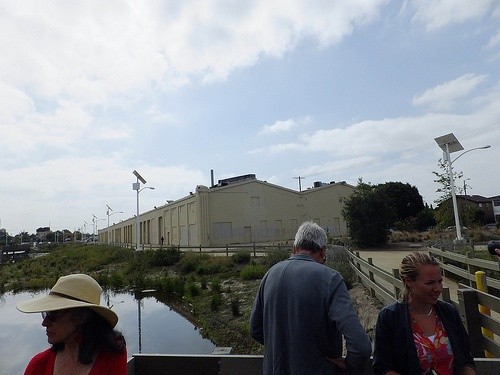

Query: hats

[15, 274, 119, 329]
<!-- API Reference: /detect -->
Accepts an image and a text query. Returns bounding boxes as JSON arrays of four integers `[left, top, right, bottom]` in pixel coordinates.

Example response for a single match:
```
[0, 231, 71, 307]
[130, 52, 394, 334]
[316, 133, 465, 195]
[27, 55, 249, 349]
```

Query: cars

[445, 225, 468, 231]
[483, 223, 496, 229]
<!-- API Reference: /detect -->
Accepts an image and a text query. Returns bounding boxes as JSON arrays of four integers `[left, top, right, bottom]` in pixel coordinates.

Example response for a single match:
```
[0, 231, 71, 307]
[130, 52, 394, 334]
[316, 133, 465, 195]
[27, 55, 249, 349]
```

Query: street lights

[108, 212, 123, 245]
[448, 145, 491, 239]
[136, 187, 155, 251]
[74, 219, 106, 242]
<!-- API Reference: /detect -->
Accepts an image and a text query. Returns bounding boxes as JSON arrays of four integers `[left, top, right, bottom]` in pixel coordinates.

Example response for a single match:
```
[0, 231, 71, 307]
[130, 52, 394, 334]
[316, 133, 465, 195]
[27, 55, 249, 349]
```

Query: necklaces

[408, 305, 433, 316]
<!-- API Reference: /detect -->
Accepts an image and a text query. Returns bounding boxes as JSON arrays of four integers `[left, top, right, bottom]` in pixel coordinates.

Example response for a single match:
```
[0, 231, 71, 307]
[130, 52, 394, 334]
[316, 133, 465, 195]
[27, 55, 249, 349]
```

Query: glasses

[41, 310, 76, 322]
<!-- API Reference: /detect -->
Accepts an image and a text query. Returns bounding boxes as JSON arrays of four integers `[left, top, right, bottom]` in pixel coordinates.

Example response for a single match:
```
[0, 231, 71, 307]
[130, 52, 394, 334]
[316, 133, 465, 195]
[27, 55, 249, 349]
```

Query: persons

[15, 273, 128, 375]
[249, 221, 373, 375]
[371, 252, 477, 375]
